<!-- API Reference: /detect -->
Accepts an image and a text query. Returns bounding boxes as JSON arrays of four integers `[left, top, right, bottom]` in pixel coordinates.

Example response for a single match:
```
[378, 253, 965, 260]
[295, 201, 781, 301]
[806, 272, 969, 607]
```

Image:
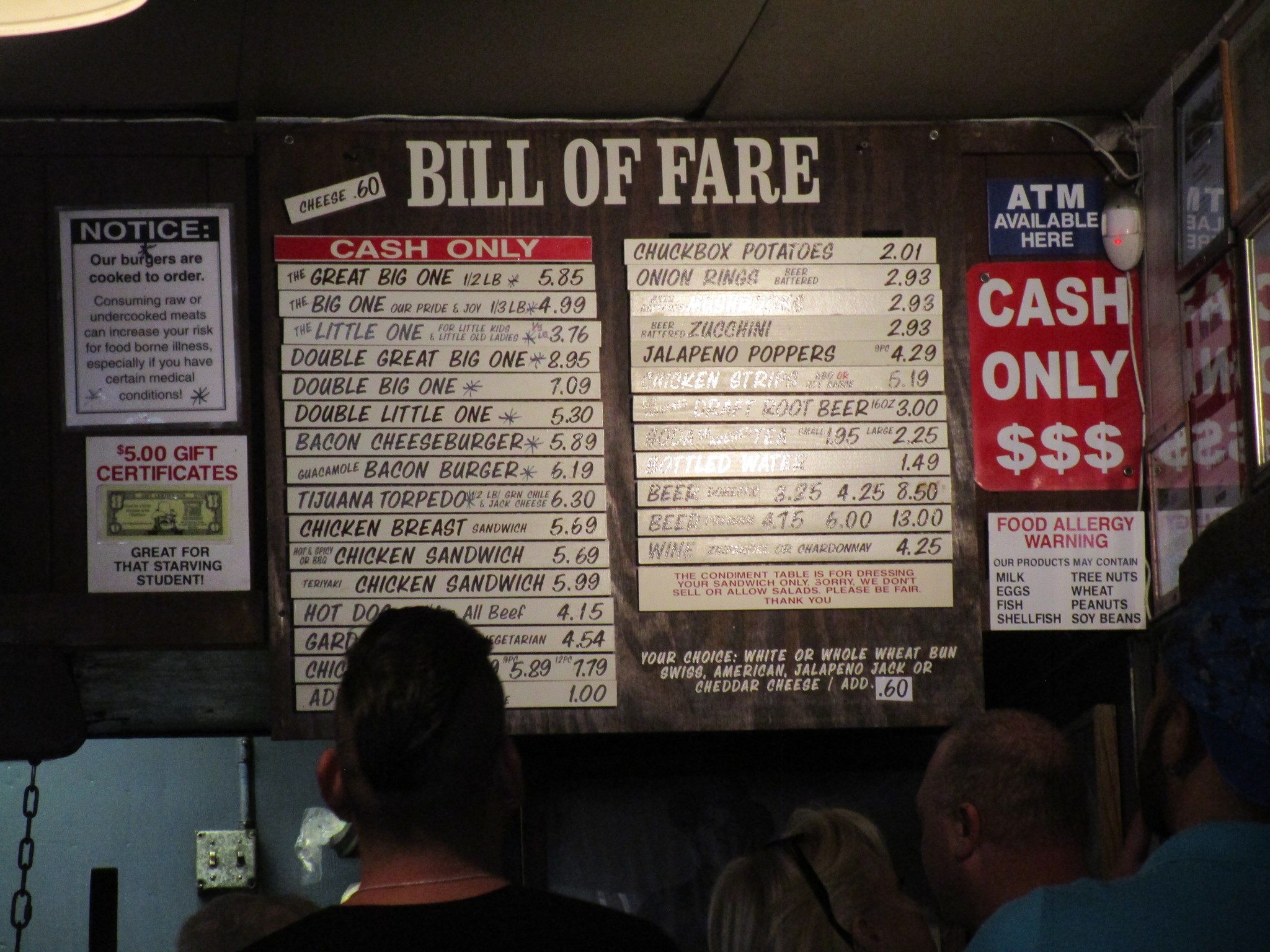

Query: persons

[962, 504, 1270, 952]
[705, 796, 939, 952]
[176, 891, 320, 952]
[239, 607, 676, 952]
[915, 707, 1098, 926]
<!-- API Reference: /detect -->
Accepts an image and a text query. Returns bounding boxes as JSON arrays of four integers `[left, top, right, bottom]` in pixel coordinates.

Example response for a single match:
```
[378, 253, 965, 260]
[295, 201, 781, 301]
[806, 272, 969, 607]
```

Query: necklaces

[359, 872, 503, 890]
